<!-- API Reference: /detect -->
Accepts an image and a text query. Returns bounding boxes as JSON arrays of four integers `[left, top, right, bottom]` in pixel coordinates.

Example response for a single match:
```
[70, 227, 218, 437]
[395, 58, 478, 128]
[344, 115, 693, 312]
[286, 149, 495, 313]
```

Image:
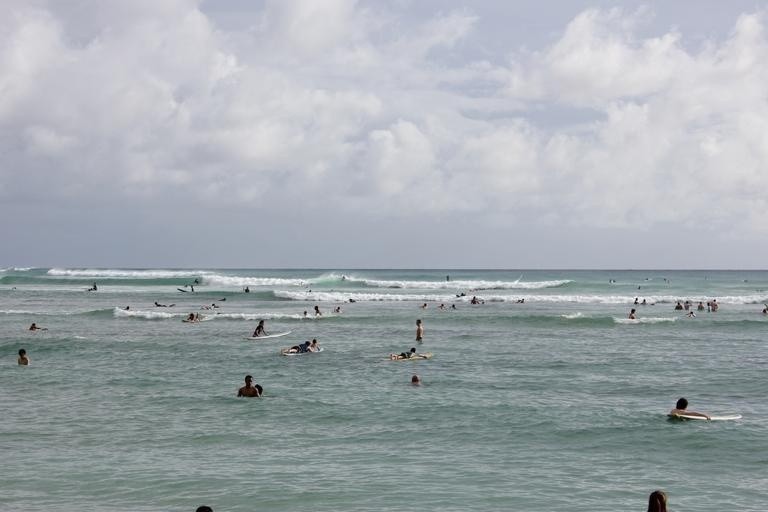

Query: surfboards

[667, 412, 745, 422]
[394, 352, 433, 361]
[241, 331, 293, 340]
[281, 346, 324, 355]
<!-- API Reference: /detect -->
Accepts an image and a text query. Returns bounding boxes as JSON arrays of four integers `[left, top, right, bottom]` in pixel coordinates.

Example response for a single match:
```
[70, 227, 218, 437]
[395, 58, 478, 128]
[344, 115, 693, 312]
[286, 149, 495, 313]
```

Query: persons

[763, 304, 768, 313]
[152, 278, 227, 322]
[412, 375, 422, 386]
[421, 276, 525, 310]
[390, 348, 428, 362]
[281, 338, 320, 355]
[237, 375, 261, 397]
[17, 348, 29, 366]
[88, 281, 97, 291]
[196, 506, 213, 512]
[647, 491, 667, 512]
[251, 320, 269, 337]
[669, 397, 711, 424]
[28, 322, 41, 331]
[301, 289, 356, 317]
[124, 306, 129, 310]
[243, 286, 249, 293]
[416, 319, 423, 343]
[609, 278, 718, 321]
